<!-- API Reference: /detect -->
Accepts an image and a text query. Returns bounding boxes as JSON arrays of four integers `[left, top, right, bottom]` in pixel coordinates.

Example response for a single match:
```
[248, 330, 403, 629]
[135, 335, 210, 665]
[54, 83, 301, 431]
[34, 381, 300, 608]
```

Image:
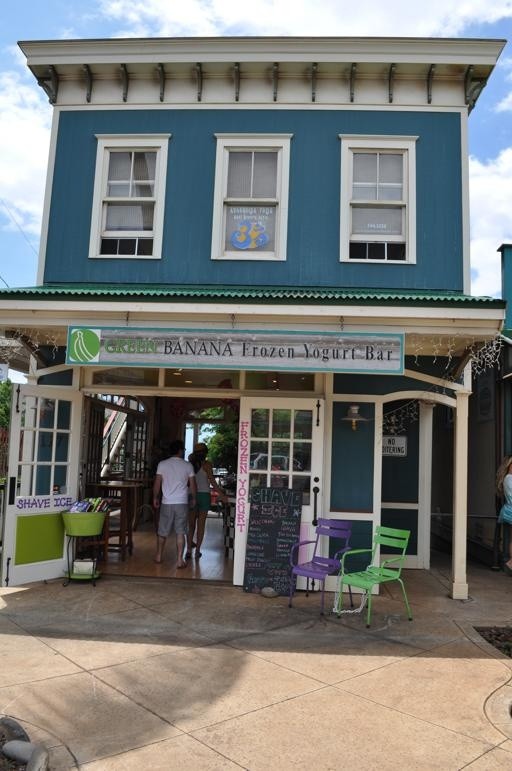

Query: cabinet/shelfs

[63, 533, 101, 588]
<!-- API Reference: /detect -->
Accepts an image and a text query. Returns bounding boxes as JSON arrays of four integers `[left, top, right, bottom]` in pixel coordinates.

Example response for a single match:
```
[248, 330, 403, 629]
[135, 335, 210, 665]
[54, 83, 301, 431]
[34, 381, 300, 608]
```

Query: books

[70, 497, 109, 513]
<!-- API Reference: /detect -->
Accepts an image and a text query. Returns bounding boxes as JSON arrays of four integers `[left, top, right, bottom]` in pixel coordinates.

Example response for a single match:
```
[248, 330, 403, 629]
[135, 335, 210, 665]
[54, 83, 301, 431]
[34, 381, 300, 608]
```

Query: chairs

[287, 518, 412, 629]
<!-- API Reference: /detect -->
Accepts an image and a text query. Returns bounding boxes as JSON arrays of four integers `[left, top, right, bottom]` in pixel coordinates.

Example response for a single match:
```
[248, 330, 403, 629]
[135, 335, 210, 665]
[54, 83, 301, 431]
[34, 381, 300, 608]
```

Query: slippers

[176, 562, 188, 569]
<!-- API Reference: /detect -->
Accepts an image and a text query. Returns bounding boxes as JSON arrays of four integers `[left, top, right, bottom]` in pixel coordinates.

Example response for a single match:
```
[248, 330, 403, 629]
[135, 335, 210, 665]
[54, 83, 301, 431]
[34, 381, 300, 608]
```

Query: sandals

[185, 551, 201, 559]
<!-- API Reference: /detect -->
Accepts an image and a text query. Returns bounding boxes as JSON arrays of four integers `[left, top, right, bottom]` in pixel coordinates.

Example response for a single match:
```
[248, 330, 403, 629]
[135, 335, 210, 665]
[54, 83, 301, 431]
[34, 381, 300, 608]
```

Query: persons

[151, 441, 227, 568]
[498, 459, 512, 571]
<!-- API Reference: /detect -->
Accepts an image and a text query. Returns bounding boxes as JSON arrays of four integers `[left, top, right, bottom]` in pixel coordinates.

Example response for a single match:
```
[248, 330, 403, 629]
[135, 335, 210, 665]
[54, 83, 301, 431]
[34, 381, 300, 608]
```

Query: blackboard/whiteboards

[243, 488, 303, 597]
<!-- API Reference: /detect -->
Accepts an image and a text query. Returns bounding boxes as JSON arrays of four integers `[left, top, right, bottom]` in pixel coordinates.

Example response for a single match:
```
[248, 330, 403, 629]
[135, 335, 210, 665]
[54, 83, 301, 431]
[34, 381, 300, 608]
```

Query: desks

[86, 480, 145, 562]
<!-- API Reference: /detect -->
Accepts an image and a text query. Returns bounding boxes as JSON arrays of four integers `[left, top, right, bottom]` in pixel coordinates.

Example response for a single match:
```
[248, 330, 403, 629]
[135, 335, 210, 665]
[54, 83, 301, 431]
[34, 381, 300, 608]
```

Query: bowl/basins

[61, 510, 106, 536]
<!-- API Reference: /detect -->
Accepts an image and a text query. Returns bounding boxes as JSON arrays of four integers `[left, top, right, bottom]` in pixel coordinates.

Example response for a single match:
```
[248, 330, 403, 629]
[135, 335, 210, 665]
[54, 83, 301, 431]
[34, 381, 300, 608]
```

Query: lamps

[341, 406, 368, 432]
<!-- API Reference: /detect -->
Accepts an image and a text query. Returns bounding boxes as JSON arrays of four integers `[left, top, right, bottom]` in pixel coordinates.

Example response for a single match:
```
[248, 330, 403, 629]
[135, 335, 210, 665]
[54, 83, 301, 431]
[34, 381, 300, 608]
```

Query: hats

[193, 442, 209, 451]
